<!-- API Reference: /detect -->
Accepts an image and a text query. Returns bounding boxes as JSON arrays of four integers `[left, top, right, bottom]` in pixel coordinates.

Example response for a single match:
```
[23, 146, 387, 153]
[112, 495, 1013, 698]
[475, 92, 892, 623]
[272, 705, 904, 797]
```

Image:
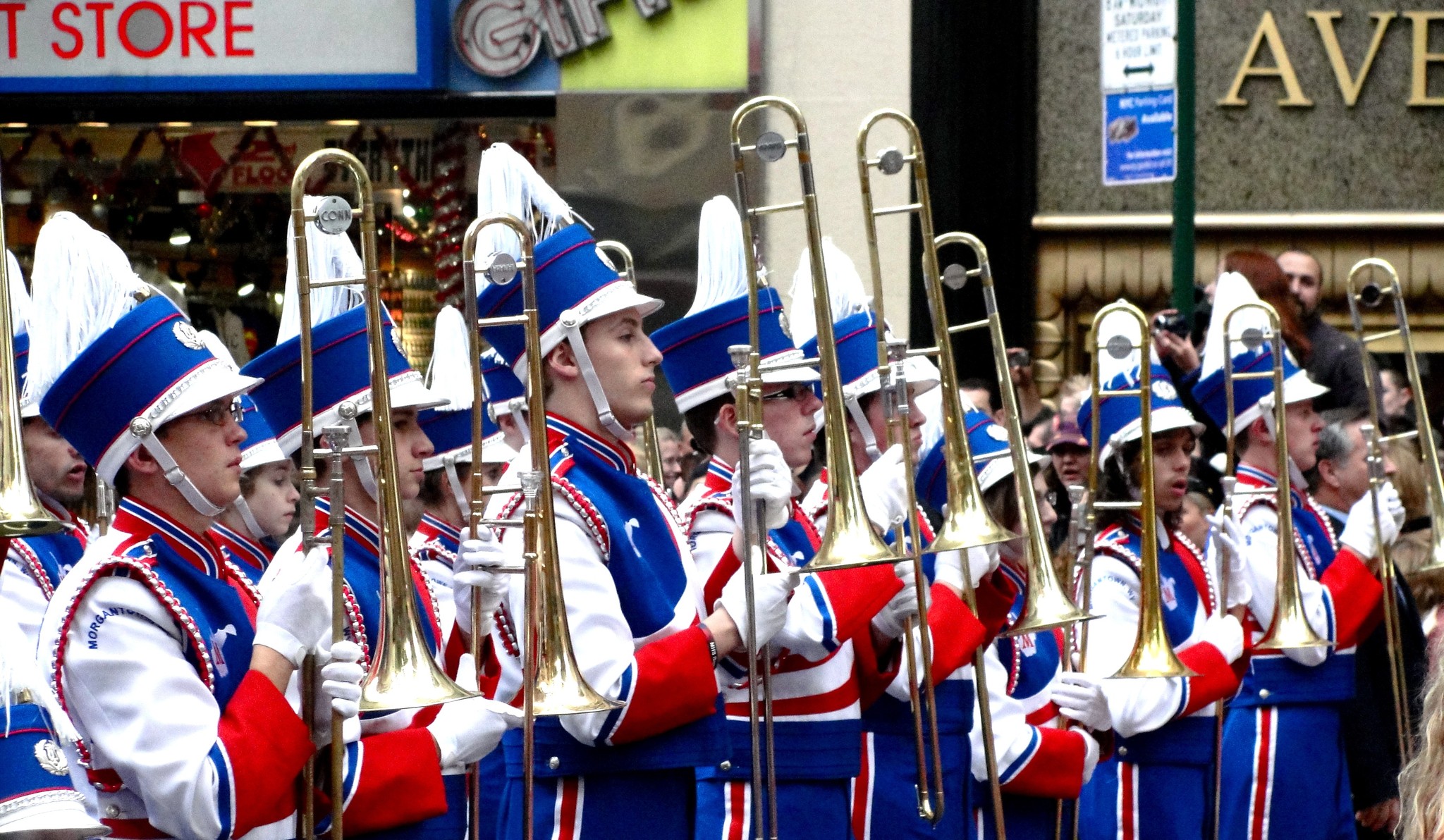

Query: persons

[1, 250, 1444, 840]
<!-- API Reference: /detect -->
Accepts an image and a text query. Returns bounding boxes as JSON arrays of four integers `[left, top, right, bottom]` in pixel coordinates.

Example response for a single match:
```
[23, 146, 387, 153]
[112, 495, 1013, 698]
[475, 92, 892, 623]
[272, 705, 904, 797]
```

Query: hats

[4, 210, 265, 491]
[0, 613, 113, 839]
[474, 140, 665, 388]
[237, 194, 520, 475]
[477, 344, 530, 423]
[648, 196, 941, 440]
[1046, 372, 1094, 453]
[913, 381, 1053, 535]
[1076, 268, 1332, 476]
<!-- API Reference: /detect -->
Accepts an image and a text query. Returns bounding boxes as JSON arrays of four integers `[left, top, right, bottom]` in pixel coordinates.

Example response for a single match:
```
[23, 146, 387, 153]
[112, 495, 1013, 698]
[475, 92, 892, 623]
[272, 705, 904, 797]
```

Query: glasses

[186, 402, 243, 425]
[761, 381, 817, 403]
[1035, 490, 1058, 507]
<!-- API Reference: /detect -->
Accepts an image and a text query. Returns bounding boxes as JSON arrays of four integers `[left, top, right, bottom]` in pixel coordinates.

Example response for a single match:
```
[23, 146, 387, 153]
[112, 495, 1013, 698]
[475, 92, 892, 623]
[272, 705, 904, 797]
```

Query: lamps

[233, 270, 255, 297]
[169, 220, 191, 246]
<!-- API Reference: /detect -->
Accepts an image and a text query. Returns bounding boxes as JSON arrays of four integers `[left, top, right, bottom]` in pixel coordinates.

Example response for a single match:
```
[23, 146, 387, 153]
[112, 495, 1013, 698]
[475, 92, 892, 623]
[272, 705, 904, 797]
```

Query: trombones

[290, 146, 484, 840]
[924, 230, 1031, 840]
[0, 206, 77, 538]
[1346, 256, 1443, 765]
[1214, 298, 1340, 840]
[460, 211, 628, 840]
[1055, 297, 1202, 840]
[592, 240, 665, 496]
[854, 105, 1059, 840]
[720, 96, 917, 840]
[92, 456, 115, 540]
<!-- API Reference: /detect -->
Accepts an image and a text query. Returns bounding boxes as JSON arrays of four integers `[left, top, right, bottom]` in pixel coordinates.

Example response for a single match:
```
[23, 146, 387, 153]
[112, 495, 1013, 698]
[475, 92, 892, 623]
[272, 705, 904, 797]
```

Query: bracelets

[696, 623, 718, 670]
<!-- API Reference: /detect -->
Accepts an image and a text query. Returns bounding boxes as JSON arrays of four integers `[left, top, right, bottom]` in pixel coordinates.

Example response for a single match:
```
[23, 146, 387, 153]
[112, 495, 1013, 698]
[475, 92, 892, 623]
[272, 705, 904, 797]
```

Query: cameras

[1154, 314, 1188, 339]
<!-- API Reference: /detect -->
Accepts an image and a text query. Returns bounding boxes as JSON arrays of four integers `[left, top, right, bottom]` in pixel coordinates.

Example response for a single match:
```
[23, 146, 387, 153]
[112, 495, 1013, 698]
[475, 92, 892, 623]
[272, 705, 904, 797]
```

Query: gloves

[933, 496, 1002, 592]
[298, 624, 365, 741]
[251, 543, 334, 669]
[1070, 724, 1100, 784]
[712, 545, 801, 658]
[1048, 668, 1115, 731]
[871, 561, 931, 639]
[730, 430, 792, 533]
[452, 524, 508, 639]
[1195, 608, 1245, 664]
[1202, 511, 1255, 607]
[426, 653, 536, 770]
[1340, 481, 1407, 559]
[857, 442, 910, 534]
